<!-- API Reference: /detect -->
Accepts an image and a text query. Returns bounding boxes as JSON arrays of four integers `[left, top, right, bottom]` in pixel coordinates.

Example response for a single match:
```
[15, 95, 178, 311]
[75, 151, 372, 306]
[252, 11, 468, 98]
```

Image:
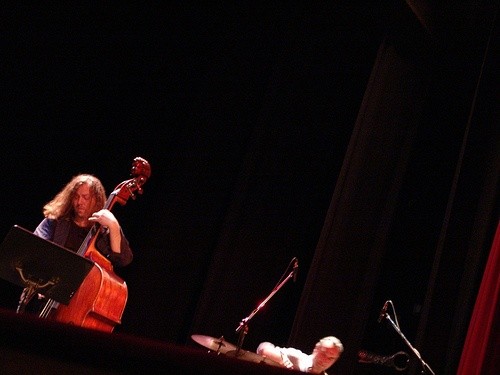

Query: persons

[256, 336, 343, 375]
[17, 174, 133, 318]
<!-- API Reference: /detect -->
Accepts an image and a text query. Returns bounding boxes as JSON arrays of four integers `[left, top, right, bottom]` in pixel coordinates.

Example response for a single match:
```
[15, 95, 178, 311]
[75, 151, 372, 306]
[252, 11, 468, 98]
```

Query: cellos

[36, 157, 151, 332]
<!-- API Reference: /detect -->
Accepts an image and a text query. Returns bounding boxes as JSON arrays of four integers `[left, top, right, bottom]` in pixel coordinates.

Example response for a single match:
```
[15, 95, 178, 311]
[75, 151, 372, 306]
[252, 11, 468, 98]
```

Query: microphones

[378, 302, 388, 324]
[292, 259, 298, 282]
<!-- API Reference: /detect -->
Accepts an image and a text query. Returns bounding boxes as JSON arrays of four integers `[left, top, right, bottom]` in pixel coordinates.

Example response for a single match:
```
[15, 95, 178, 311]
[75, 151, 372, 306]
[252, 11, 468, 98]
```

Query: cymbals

[224, 351, 279, 366]
[191, 335, 237, 353]
